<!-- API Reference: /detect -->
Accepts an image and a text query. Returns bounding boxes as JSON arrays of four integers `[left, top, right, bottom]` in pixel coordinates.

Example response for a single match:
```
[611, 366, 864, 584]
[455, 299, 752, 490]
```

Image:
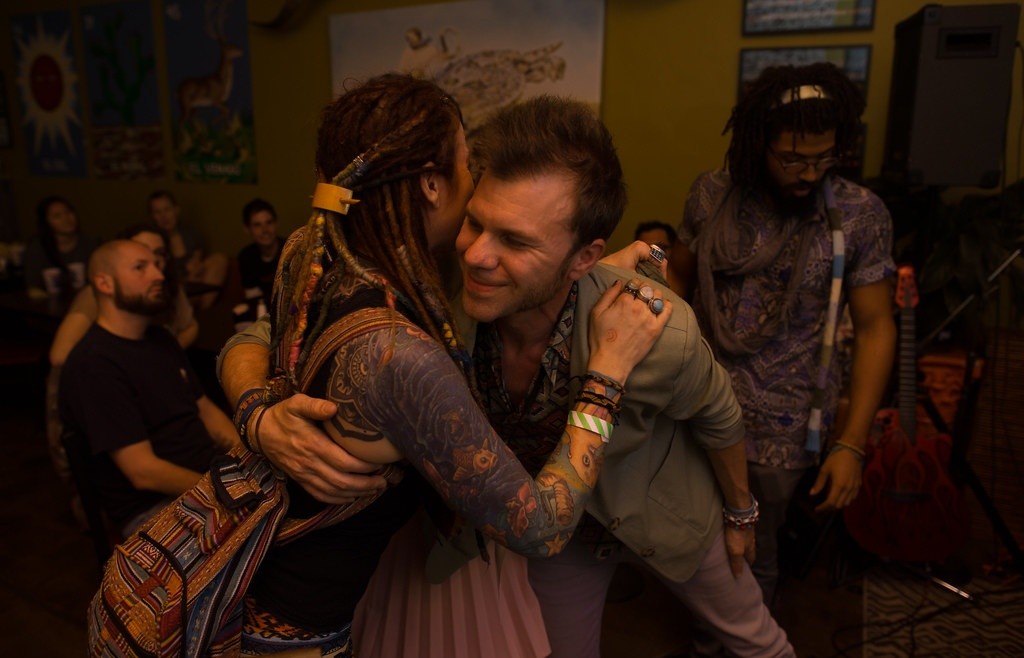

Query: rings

[648, 297, 664, 313]
[647, 242, 666, 264]
[637, 286, 653, 302]
[623, 281, 639, 295]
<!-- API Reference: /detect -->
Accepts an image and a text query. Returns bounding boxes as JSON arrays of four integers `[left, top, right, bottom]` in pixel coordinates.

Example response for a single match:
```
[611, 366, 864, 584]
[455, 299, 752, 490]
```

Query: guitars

[842, 264, 965, 574]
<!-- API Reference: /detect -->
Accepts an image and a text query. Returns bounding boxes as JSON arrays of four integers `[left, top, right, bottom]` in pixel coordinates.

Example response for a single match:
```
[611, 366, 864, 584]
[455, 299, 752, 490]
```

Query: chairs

[45, 358, 125, 552]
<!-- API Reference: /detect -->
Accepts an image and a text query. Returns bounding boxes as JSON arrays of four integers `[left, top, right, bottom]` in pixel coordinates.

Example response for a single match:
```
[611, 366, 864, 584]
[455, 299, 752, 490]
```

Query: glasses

[766, 143, 843, 176]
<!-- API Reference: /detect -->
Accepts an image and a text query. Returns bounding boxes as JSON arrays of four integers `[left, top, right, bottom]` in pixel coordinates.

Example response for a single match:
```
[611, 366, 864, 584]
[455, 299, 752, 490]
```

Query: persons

[58, 239, 243, 541]
[87, 71, 673, 658]
[224, 198, 291, 289]
[34, 189, 228, 368]
[634, 220, 677, 254]
[668, 62, 897, 658]
[215, 92, 796, 658]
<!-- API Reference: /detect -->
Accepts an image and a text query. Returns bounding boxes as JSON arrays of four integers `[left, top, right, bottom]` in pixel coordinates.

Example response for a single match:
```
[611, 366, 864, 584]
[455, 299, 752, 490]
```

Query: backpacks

[85, 307, 425, 658]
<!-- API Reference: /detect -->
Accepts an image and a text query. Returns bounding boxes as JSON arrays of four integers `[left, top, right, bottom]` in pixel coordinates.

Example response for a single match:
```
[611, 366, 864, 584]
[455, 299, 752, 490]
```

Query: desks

[0, 280, 222, 341]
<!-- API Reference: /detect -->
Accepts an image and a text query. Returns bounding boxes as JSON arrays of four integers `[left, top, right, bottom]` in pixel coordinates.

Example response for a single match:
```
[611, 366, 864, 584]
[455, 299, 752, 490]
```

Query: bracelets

[835, 440, 866, 457]
[563, 373, 627, 443]
[232, 389, 281, 454]
[722, 492, 760, 531]
